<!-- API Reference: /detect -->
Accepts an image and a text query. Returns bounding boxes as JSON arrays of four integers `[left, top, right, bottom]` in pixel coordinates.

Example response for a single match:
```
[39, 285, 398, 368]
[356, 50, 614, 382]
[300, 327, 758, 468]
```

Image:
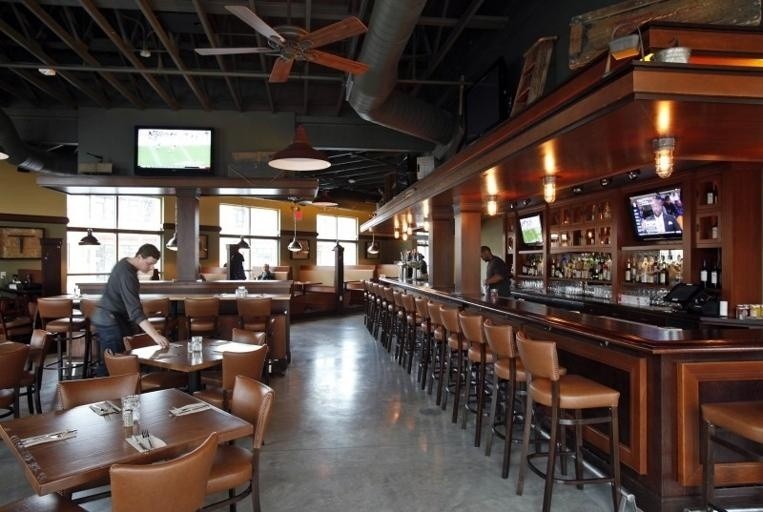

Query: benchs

[251, 263, 400, 315]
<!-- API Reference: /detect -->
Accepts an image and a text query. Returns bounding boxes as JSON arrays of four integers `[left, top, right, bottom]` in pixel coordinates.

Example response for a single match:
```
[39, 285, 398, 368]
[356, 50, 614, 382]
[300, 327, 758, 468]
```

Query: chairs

[204, 375, 274, 511]
[0, 491, 87, 511]
[110, 431, 217, 510]
[0, 294, 290, 447]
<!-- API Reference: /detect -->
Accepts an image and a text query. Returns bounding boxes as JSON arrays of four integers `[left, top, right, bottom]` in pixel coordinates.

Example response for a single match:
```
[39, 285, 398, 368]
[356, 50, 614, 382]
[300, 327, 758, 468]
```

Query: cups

[120, 395, 140, 420]
[489, 288, 498, 297]
[234, 286, 248, 298]
[520, 279, 612, 299]
[719, 300, 728, 317]
[191, 336, 201, 351]
[630, 287, 671, 305]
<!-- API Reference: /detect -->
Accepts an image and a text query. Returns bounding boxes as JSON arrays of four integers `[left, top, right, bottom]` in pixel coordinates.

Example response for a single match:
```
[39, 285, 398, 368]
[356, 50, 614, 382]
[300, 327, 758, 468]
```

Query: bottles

[121, 408, 132, 427]
[700, 183, 717, 206]
[520, 253, 612, 281]
[711, 217, 717, 239]
[625, 255, 682, 284]
[735, 303, 763, 320]
[699, 257, 721, 289]
[186, 341, 193, 353]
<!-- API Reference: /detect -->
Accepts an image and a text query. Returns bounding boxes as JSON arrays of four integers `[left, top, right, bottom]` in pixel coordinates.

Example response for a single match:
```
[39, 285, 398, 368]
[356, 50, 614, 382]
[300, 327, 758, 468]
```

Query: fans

[194, 1, 367, 89]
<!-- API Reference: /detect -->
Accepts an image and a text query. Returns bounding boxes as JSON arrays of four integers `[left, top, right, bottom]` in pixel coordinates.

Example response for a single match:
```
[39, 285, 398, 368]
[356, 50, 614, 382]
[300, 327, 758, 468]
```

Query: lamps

[539, 175, 558, 205]
[165, 232, 177, 252]
[486, 194, 498, 217]
[390, 221, 430, 242]
[76, 195, 101, 246]
[285, 195, 303, 252]
[650, 136, 674, 179]
[265, 98, 333, 174]
[506, 166, 642, 212]
[366, 229, 378, 254]
[312, 179, 339, 207]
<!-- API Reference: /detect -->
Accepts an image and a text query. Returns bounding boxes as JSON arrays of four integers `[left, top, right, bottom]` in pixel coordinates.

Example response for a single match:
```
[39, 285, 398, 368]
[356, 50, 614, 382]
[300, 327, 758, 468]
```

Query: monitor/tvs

[516, 212, 544, 247]
[624, 183, 683, 242]
[133, 125, 215, 177]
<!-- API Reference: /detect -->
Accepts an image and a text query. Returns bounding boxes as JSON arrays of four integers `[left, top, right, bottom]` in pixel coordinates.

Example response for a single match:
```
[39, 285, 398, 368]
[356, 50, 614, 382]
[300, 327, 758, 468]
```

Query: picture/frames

[289, 239, 310, 259]
[198, 234, 208, 260]
[364, 240, 380, 259]
[0, 225, 45, 261]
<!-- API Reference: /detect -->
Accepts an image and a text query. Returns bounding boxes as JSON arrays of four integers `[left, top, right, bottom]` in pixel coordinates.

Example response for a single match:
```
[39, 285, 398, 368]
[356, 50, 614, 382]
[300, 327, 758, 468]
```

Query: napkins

[126, 434, 167, 453]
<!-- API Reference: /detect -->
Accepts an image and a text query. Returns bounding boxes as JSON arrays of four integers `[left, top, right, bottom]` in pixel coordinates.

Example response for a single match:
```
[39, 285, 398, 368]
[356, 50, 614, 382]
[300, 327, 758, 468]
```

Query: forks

[168, 403, 209, 416]
[141, 429, 151, 449]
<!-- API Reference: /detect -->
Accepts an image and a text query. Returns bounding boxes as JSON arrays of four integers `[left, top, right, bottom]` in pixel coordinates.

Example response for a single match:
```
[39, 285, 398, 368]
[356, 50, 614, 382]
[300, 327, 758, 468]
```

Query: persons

[92, 244, 170, 376]
[253, 264, 273, 279]
[641, 196, 683, 233]
[417, 254, 428, 279]
[480, 246, 510, 298]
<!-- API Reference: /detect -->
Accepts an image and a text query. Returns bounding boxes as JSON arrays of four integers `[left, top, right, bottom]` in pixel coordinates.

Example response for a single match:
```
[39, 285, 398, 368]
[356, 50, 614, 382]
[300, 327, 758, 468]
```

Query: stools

[483, 319, 566, 479]
[701, 395, 762, 509]
[356, 275, 526, 448]
[515, 332, 621, 511]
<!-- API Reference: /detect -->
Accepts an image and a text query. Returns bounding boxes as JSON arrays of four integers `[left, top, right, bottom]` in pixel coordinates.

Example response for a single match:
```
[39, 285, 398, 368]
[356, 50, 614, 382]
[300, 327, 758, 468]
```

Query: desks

[0, 388, 253, 506]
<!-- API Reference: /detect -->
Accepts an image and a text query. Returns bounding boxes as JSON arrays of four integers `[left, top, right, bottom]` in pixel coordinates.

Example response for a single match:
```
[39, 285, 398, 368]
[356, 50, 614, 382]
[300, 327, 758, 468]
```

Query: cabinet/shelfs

[503, 162, 761, 317]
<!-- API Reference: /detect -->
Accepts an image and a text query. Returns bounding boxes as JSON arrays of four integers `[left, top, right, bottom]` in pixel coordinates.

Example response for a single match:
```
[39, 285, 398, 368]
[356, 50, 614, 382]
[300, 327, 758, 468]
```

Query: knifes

[131, 435, 148, 452]
[105, 399, 119, 413]
[20, 429, 76, 442]
